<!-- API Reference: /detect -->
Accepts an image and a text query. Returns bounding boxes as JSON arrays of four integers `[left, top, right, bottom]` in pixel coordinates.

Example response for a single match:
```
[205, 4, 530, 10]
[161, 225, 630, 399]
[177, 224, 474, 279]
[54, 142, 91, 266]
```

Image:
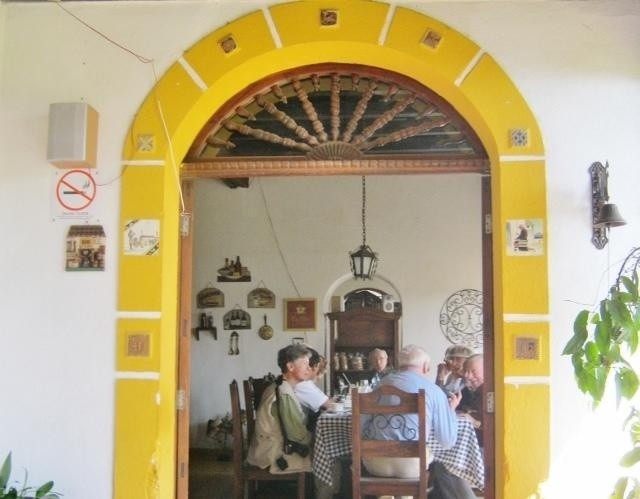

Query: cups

[327, 403, 344, 413]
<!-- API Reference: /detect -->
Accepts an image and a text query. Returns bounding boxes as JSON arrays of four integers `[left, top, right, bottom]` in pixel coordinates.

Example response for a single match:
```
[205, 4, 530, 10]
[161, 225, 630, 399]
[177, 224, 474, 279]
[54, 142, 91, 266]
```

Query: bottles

[225, 256, 242, 277]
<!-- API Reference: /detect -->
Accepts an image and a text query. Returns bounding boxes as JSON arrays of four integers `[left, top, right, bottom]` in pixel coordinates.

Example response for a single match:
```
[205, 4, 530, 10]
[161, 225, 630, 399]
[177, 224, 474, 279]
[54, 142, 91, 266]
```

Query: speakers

[43, 101, 98, 170]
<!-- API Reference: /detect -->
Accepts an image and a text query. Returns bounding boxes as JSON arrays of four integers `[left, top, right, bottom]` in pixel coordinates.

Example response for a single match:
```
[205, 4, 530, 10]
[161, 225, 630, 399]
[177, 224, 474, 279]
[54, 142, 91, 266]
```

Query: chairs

[229, 377, 426, 498]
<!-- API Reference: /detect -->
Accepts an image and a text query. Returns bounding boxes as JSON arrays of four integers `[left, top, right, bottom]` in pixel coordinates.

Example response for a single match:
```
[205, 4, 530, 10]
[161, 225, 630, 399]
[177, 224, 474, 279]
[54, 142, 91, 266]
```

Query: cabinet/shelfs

[326, 310, 400, 397]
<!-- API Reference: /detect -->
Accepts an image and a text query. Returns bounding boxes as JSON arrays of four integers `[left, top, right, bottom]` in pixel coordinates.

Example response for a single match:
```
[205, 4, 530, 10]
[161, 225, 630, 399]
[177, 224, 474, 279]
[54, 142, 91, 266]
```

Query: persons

[431, 347, 483, 499]
[365, 349, 395, 384]
[360, 344, 463, 498]
[246, 341, 343, 498]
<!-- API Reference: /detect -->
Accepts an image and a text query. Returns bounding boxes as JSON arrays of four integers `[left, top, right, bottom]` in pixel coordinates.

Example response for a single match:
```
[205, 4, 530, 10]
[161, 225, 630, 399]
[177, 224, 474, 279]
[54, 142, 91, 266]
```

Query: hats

[445, 345, 475, 361]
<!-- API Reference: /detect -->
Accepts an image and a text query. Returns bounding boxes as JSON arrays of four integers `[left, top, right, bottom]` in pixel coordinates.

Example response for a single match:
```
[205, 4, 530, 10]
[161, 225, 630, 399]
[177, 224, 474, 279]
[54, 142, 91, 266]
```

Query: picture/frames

[282, 297, 317, 332]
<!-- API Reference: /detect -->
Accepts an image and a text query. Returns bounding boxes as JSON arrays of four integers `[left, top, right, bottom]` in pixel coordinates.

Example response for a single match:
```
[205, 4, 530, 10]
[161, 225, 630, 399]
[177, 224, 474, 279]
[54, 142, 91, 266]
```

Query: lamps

[348, 174, 378, 280]
